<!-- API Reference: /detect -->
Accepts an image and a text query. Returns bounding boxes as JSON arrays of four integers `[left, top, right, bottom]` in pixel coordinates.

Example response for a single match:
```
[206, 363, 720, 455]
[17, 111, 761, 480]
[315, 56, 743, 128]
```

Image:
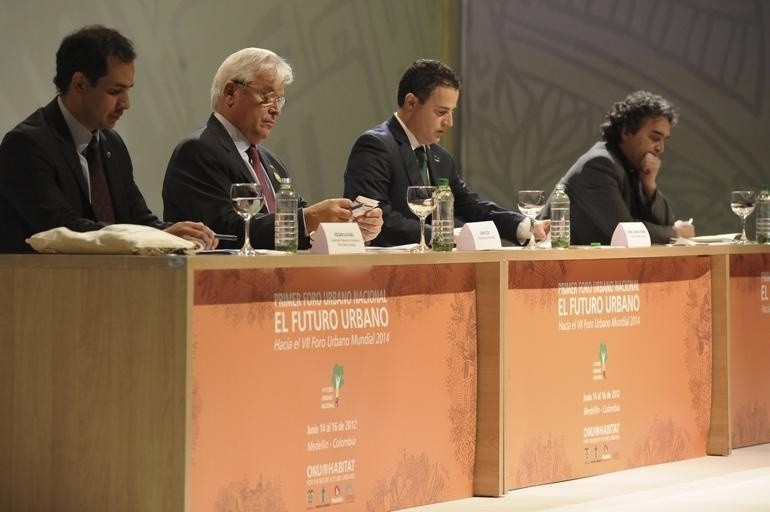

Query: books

[668, 231, 743, 244]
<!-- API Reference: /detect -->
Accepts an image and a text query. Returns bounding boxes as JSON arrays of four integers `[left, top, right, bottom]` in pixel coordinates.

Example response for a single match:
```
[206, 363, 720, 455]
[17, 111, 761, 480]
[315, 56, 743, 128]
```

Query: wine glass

[730, 189, 757, 246]
[516, 189, 548, 251]
[229, 182, 265, 256]
[406, 185, 440, 254]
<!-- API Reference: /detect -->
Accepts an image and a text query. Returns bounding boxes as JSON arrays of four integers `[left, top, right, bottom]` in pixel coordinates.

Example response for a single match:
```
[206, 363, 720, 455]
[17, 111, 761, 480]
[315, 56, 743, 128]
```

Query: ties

[414, 146, 433, 198]
[81, 136, 115, 225]
[245, 145, 277, 217]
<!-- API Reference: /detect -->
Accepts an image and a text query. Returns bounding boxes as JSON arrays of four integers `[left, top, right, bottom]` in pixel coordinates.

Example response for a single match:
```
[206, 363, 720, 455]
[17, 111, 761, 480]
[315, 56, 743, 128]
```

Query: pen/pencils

[688, 218, 694, 224]
[350, 204, 363, 210]
[214, 234, 237, 239]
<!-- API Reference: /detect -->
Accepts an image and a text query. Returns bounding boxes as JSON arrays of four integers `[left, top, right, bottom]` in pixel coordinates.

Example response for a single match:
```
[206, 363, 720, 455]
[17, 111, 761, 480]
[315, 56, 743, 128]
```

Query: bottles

[755, 181, 770, 244]
[549, 184, 573, 250]
[430, 178, 456, 253]
[273, 177, 300, 253]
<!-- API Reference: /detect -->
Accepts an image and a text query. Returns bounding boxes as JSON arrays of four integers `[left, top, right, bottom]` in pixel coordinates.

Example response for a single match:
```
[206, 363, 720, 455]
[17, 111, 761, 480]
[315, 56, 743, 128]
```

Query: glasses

[234, 78, 288, 108]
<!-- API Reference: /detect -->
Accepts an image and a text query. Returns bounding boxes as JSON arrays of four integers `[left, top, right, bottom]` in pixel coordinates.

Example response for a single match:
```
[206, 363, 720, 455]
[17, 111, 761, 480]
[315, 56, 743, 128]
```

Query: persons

[536, 90, 695, 245]
[341, 58, 566, 249]
[1, 25, 220, 252]
[160, 46, 386, 251]
